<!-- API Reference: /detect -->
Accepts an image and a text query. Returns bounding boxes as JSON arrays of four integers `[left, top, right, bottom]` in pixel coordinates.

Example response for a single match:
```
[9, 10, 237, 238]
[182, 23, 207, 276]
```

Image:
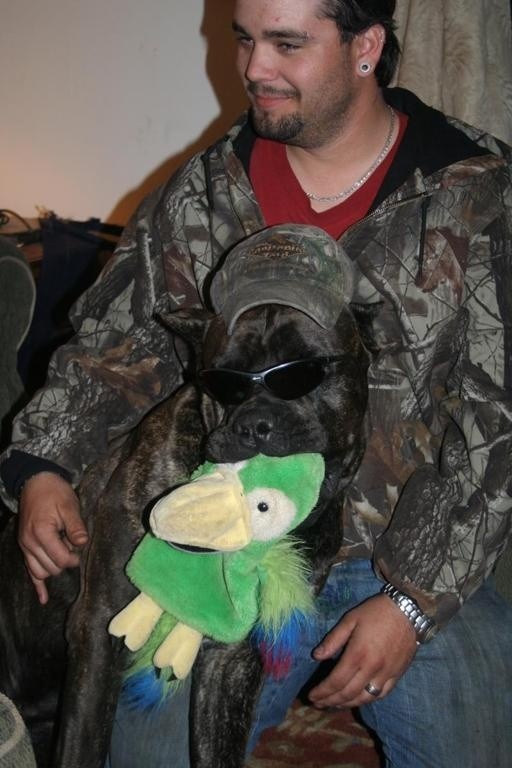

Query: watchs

[379, 581, 440, 645]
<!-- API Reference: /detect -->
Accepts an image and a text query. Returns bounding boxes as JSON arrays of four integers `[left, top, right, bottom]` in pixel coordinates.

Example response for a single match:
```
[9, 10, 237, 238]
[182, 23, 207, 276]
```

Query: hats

[209, 223, 355, 336]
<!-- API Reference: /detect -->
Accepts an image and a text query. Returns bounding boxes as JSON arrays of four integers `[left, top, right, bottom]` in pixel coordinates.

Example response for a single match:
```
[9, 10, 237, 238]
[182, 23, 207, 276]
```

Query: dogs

[0, 295, 386, 768]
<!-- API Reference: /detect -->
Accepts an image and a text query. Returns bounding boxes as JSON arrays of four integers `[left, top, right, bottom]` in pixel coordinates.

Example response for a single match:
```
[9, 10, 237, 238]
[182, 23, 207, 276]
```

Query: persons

[1, 2, 512, 767]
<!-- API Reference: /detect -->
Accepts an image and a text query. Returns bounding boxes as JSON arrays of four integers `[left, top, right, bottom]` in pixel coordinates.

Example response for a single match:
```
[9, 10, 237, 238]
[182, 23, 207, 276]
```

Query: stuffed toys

[106, 448, 328, 681]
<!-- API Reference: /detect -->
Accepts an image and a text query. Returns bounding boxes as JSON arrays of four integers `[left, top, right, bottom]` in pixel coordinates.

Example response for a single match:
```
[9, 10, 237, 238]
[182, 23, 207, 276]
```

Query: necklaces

[306, 104, 396, 202]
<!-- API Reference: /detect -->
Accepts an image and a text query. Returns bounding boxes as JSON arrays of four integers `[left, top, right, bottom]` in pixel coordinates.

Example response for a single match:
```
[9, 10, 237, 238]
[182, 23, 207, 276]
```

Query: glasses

[193, 341, 360, 405]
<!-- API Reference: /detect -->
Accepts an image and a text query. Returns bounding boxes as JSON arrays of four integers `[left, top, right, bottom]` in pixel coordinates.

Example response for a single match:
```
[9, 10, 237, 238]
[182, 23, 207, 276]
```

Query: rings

[364, 682, 383, 698]
